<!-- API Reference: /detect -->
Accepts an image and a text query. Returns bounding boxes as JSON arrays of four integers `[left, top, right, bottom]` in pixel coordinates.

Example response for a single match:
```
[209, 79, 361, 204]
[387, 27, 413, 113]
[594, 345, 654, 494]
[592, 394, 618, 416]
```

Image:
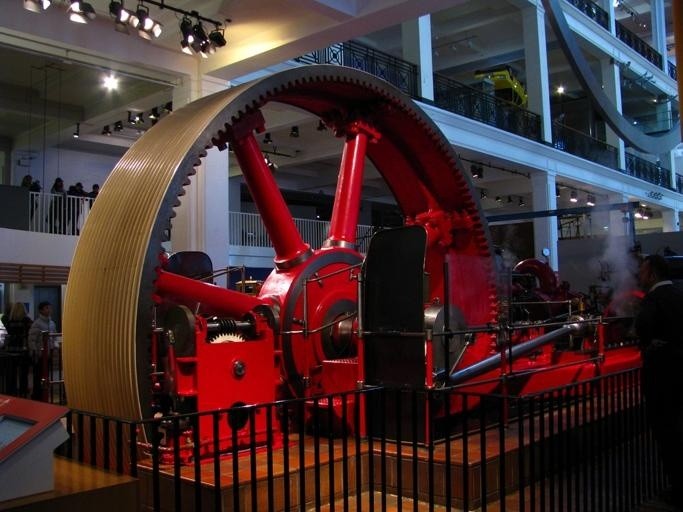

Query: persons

[21, 174, 42, 221]
[634, 255, 683, 511]
[47, 177, 99, 236]
[0, 301, 58, 402]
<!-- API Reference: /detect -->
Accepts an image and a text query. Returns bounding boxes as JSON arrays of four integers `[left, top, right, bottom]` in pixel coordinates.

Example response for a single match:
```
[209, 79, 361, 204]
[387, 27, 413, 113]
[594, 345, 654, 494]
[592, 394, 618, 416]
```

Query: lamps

[634, 208, 650, 220]
[289, 125, 299, 137]
[24, 0, 225, 59]
[556, 182, 594, 206]
[493, 195, 525, 207]
[72, 123, 79, 138]
[468, 164, 483, 180]
[261, 132, 273, 144]
[101, 102, 171, 135]
